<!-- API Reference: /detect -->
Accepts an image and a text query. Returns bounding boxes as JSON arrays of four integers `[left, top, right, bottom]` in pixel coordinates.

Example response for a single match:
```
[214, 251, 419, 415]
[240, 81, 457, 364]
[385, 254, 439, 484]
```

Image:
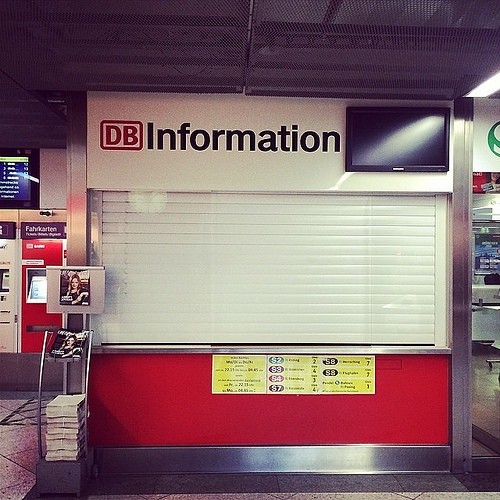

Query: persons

[484, 171, 500, 194]
[67, 274, 89, 306]
[59, 334, 77, 359]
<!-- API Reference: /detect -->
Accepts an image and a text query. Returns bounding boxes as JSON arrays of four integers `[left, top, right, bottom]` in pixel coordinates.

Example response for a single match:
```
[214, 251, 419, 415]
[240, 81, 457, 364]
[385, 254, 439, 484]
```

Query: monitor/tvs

[345, 106, 450, 172]
[0, 148, 39, 209]
